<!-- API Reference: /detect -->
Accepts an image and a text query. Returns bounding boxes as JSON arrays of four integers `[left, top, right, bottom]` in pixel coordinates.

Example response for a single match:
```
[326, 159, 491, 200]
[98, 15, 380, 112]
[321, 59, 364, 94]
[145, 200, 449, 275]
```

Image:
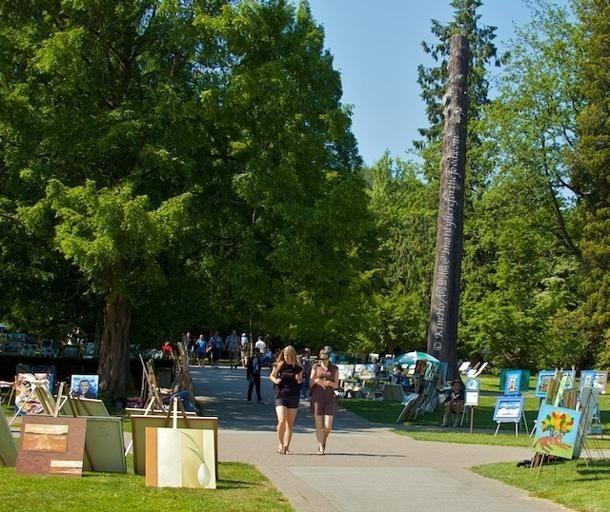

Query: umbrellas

[395, 351, 441, 393]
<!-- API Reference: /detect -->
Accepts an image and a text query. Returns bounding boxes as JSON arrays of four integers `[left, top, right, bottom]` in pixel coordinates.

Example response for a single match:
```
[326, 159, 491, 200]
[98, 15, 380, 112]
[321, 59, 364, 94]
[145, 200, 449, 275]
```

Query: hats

[451, 379, 462, 386]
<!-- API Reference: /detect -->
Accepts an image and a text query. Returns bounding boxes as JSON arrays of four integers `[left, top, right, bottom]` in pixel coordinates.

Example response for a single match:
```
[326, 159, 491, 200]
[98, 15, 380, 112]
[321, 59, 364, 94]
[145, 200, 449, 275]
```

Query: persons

[73, 379, 96, 399]
[300, 348, 311, 402]
[309, 353, 340, 455]
[392, 365, 402, 383]
[324, 345, 337, 364]
[269, 346, 304, 455]
[184, 330, 272, 404]
[442, 378, 465, 428]
